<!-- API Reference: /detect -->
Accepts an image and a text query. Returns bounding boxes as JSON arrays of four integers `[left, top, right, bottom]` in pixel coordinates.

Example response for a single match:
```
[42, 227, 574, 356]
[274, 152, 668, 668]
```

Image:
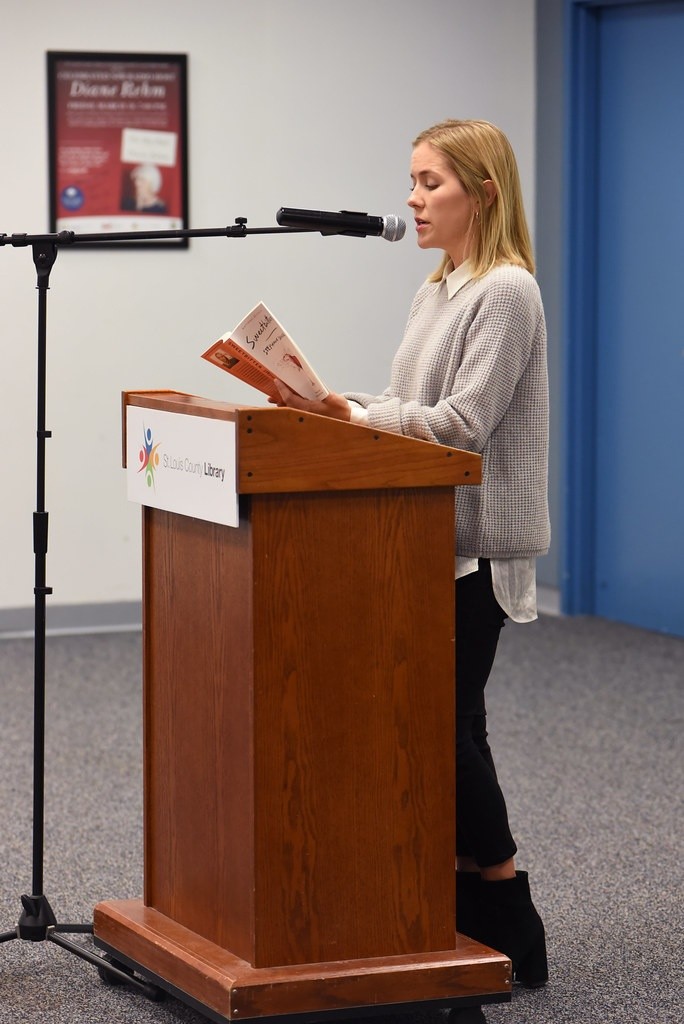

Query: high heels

[456, 866, 549, 988]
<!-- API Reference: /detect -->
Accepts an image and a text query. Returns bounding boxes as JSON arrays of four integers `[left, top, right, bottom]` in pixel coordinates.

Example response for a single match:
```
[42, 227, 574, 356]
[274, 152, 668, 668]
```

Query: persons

[267, 118, 553, 993]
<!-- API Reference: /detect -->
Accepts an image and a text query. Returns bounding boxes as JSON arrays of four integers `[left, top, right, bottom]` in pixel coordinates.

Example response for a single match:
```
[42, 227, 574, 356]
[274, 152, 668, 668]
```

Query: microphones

[277, 207, 406, 242]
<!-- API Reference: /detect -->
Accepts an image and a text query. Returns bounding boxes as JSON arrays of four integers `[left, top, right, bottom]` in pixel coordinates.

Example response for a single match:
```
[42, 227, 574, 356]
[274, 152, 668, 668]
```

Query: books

[201, 302, 329, 403]
